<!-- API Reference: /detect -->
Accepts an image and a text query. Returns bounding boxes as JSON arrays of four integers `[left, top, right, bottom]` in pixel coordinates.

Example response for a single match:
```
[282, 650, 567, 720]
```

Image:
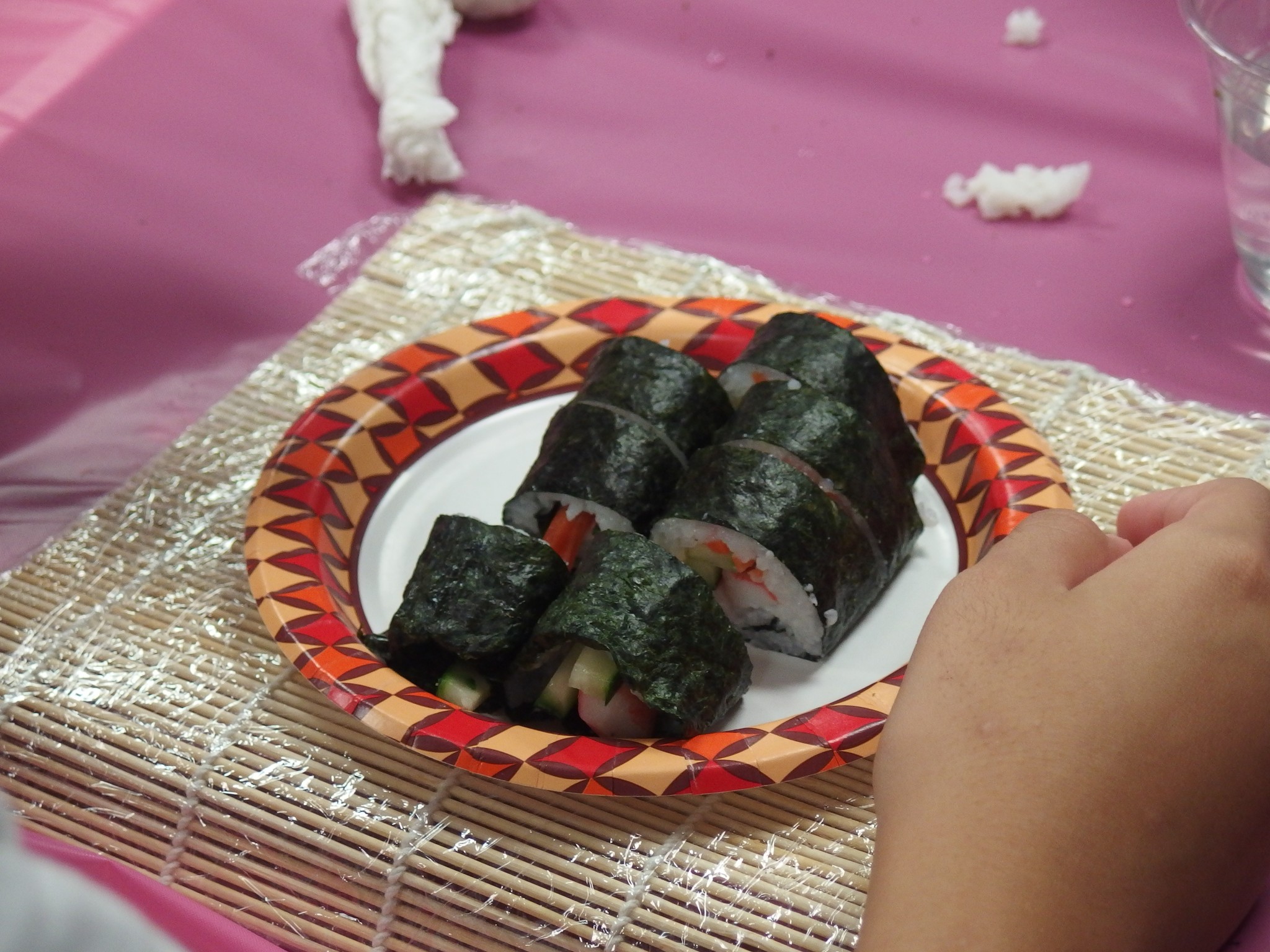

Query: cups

[1173, 1, 1270, 319]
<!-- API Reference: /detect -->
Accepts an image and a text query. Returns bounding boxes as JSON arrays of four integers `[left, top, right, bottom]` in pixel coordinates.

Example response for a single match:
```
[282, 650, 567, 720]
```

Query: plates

[243, 296, 1078, 798]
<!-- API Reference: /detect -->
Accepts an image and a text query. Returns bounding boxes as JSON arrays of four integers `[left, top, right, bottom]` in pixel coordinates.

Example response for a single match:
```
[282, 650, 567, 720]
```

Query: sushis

[364, 314, 924, 739]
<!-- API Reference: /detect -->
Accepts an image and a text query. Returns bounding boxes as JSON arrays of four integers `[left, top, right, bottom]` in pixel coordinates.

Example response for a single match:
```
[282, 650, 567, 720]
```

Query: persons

[854, 478, 1270, 952]
